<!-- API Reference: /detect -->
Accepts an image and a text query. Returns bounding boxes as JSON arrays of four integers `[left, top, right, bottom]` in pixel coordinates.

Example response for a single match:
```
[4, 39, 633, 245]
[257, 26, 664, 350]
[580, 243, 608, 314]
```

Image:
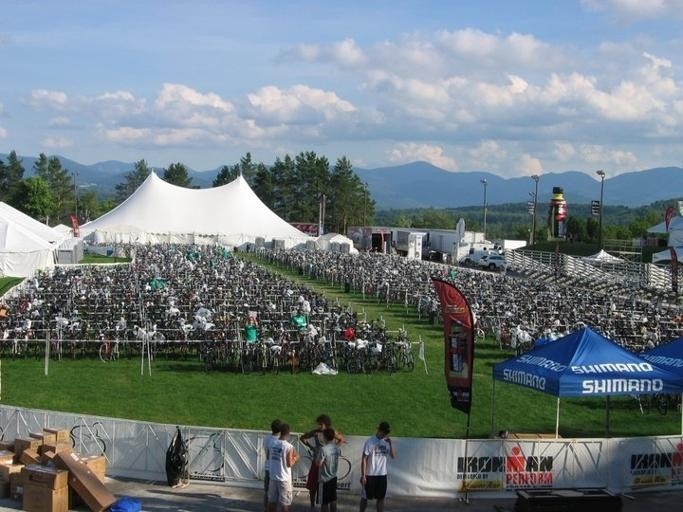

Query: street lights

[70, 170, 79, 216]
[360, 181, 369, 226]
[596, 170, 606, 250]
[480, 178, 488, 237]
[530, 174, 540, 244]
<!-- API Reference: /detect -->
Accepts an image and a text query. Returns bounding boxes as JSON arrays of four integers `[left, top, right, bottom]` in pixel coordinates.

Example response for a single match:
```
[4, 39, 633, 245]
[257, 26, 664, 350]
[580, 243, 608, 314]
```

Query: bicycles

[631, 392, 680, 416]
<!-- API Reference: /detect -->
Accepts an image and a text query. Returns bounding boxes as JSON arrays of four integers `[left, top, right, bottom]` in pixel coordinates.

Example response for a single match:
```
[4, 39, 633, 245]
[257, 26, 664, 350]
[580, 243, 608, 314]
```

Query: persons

[0, 243, 385, 352]
[267, 423, 300, 512]
[299, 413, 344, 512]
[315, 427, 341, 512]
[359, 421, 397, 512]
[262, 419, 282, 512]
[261, 248, 681, 351]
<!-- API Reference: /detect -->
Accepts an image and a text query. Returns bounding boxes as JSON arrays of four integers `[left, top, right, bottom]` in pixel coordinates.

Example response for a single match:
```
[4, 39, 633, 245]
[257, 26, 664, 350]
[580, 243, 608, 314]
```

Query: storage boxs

[1, 427, 117, 512]
